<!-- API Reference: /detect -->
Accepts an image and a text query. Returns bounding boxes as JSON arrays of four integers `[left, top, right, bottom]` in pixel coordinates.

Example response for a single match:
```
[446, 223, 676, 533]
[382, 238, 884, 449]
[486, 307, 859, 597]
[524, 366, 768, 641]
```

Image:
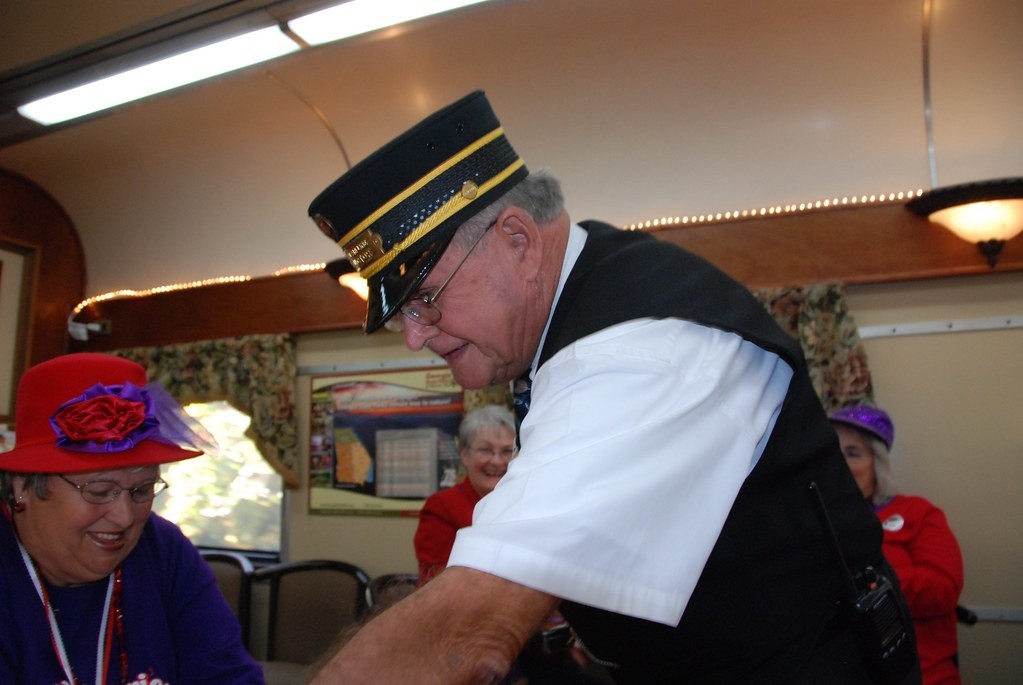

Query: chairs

[196, 549, 253, 656]
[364, 574, 420, 617]
[253, 559, 371, 666]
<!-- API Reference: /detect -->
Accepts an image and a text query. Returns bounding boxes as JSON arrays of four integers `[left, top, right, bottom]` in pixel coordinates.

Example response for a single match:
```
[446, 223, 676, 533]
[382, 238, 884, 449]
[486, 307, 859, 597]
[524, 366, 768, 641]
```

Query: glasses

[398, 218, 498, 327]
[470, 444, 515, 461]
[59, 473, 170, 503]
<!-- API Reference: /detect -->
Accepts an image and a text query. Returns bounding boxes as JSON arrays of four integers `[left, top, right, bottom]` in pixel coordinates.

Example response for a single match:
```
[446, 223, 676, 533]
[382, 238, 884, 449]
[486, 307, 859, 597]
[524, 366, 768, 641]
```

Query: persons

[307, 87, 924, 685]
[0, 353, 266, 685]
[413, 403, 519, 589]
[827, 406, 964, 685]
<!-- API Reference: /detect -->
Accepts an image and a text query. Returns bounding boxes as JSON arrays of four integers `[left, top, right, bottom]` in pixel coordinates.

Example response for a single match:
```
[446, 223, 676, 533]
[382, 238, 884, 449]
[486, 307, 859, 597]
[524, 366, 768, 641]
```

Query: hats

[0, 354, 204, 472]
[827, 402, 894, 451]
[310, 93, 525, 333]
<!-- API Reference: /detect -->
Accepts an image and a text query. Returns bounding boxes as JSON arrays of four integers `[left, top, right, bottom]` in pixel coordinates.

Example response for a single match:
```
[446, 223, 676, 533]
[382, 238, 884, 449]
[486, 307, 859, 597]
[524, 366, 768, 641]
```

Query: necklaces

[2, 502, 122, 685]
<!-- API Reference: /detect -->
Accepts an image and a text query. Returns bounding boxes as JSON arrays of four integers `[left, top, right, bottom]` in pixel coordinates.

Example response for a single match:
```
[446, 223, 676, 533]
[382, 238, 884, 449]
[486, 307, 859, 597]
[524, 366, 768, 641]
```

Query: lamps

[903, 176, 1023, 271]
[324, 257, 369, 305]
